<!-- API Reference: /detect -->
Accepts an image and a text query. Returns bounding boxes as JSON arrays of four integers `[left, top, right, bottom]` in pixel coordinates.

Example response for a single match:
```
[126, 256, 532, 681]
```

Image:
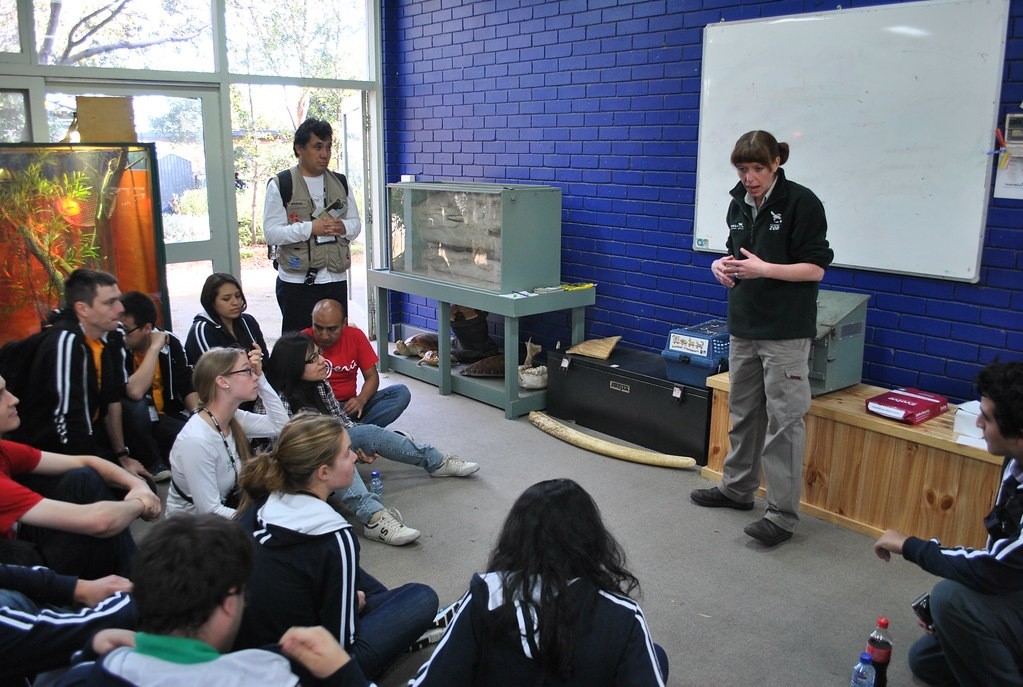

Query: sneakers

[429, 454, 480, 477]
[363, 507, 422, 545]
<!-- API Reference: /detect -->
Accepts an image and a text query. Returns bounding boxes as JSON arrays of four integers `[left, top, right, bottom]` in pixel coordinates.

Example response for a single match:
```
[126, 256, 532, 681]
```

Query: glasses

[305, 347, 323, 364]
[221, 367, 255, 376]
[125, 327, 139, 336]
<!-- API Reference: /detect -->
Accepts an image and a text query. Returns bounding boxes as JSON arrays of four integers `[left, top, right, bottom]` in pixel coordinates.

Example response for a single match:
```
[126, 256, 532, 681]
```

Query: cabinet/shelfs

[367, 264, 596, 421]
[701, 370, 1006, 551]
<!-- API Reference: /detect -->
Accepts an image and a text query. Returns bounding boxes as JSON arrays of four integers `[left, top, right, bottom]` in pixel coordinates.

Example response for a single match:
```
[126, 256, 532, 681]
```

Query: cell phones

[912, 592, 935, 633]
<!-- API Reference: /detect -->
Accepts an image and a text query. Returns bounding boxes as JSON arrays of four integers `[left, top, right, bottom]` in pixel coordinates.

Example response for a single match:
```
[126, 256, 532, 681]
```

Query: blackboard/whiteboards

[692, 0, 1010, 285]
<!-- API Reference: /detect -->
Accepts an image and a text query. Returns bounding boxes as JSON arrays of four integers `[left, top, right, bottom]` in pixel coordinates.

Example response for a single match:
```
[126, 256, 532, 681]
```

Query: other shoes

[401, 600, 458, 652]
[745, 519, 792, 545]
[395, 430, 414, 441]
[690, 487, 754, 510]
[146, 463, 171, 481]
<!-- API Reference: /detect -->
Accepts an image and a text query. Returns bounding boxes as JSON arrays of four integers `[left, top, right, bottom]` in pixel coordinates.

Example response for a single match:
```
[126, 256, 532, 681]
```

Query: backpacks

[0, 319, 81, 398]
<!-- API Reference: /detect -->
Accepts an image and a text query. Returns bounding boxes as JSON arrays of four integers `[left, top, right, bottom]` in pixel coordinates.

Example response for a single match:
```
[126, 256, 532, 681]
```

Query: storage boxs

[953, 399, 985, 439]
[546, 344, 729, 467]
[385, 181, 562, 295]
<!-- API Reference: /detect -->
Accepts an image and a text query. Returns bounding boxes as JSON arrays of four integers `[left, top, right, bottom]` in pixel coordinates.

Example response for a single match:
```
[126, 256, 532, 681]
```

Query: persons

[52, 513, 378, 687]
[0, 561, 139, 687]
[16, 269, 480, 544]
[233, 412, 438, 679]
[874, 361, 1023, 687]
[163, 198, 178, 215]
[408, 478, 669, 687]
[690, 130, 834, 545]
[263, 117, 362, 335]
[0, 376, 161, 581]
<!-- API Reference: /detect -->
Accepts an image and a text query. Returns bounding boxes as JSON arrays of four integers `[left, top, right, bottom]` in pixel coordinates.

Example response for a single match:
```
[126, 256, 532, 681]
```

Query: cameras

[304, 268, 319, 286]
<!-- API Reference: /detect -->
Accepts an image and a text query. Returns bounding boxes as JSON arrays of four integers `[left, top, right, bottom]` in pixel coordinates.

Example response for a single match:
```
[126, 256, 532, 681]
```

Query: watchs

[117, 446, 130, 458]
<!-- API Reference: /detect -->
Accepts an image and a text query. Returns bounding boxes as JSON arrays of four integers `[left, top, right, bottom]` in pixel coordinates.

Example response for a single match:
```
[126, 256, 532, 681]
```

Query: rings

[735, 272, 738, 276]
